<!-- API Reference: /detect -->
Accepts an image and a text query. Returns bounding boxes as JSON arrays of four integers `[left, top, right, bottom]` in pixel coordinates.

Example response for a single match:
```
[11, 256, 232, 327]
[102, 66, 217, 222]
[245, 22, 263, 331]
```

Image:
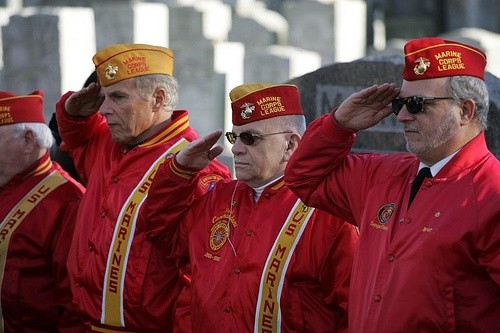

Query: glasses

[390, 96, 468, 114]
[226, 131, 292, 145]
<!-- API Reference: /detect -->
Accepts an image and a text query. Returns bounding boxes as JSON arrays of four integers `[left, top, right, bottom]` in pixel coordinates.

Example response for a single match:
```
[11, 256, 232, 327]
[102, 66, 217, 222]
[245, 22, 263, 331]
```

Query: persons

[0, 89, 88, 333]
[136, 83, 360, 333]
[284, 38, 500, 332]
[56, 43, 234, 332]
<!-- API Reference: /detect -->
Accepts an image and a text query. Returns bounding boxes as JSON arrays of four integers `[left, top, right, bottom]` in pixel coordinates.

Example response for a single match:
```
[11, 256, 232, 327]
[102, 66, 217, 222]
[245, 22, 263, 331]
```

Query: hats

[402, 37, 486, 80]
[91, 44, 173, 85]
[0, 91, 46, 124]
[230, 83, 304, 124]
